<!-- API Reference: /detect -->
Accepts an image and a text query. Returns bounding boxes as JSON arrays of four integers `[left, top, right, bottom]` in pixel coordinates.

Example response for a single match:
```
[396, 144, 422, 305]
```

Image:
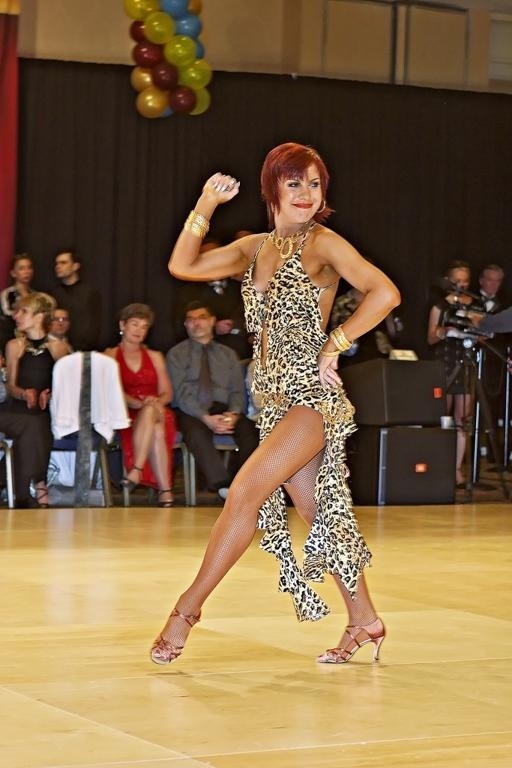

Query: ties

[196, 343, 214, 406]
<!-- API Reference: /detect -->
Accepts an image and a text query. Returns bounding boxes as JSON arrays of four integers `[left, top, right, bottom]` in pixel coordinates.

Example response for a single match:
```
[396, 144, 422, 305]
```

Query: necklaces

[22, 338, 49, 355]
[270, 230, 301, 259]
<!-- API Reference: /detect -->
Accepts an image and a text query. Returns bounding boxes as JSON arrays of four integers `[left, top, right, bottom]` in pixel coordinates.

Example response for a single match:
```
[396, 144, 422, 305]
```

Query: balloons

[124, 0, 213, 119]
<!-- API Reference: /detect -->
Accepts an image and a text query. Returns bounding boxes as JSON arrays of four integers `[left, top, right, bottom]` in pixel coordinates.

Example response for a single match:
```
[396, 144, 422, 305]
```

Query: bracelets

[21, 389, 25, 401]
[338, 325, 353, 347]
[329, 331, 345, 352]
[319, 350, 340, 356]
[184, 210, 211, 239]
[333, 329, 350, 350]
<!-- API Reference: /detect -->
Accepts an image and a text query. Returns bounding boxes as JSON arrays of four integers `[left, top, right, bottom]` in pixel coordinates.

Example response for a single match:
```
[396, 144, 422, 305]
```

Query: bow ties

[483, 295, 497, 304]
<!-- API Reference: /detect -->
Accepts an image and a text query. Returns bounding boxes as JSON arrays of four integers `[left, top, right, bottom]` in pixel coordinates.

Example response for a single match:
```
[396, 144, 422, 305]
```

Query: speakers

[339, 358, 445, 422]
[348, 424, 458, 506]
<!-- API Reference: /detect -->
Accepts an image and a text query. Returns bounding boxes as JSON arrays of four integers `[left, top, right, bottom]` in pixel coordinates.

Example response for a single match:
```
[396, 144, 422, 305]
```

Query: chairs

[1, 429, 241, 508]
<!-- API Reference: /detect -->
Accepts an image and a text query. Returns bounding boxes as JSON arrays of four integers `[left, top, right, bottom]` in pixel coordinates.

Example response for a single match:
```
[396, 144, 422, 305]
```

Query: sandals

[32, 485, 49, 507]
[118, 464, 146, 495]
[149, 599, 202, 666]
[455, 467, 468, 491]
[157, 484, 176, 506]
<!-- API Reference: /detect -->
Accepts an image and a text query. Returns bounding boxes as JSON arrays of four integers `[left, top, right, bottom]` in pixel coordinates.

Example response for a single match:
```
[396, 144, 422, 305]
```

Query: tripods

[446, 345, 508, 500]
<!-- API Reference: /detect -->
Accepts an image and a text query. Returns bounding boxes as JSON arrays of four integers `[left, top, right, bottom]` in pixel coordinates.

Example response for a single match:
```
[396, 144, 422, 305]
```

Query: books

[464, 308, 511, 333]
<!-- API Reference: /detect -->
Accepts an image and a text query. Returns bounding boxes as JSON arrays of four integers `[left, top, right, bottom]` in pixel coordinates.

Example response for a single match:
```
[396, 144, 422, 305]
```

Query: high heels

[315, 616, 388, 667]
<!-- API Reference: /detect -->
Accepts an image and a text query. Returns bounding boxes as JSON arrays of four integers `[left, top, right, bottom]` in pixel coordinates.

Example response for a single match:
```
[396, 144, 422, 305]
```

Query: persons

[427, 261, 488, 489]
[164, 300, 245, 509]
[46, 248, 102, 353]
[1, 291, 67, 508]
[151, 141, 402, 665]
[474, 264, 511, 464]
[44, 308, 74, 355]
[103, 303, 177, 509]
[171, 233, 255, 358]
[0, 252, 38, 366]
[325, 256, 402, 369]
